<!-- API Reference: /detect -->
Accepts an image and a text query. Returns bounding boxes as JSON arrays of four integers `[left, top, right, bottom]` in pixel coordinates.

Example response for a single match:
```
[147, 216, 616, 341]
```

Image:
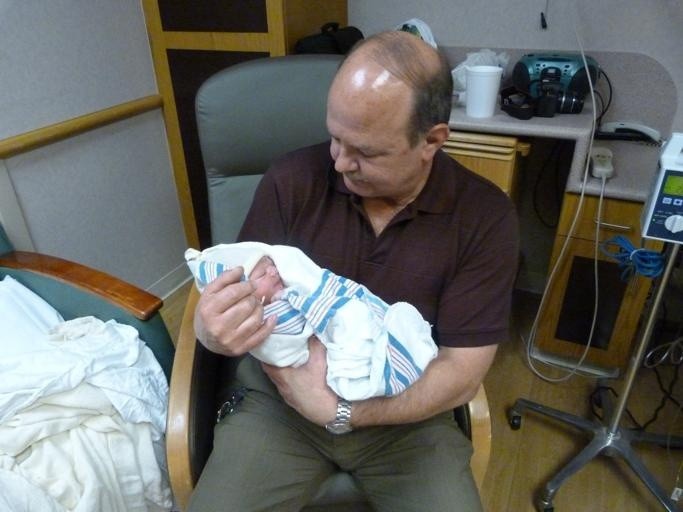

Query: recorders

[513, 53, 600, 99]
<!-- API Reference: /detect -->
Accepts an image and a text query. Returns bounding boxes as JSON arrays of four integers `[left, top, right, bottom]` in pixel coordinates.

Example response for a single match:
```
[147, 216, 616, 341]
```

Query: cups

[465, 65, 503, 119]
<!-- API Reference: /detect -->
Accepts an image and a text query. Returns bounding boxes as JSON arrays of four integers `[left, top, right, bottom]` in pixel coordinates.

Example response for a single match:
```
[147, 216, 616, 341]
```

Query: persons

[174, 25, 522, 512]
[182, 239, 437, 403]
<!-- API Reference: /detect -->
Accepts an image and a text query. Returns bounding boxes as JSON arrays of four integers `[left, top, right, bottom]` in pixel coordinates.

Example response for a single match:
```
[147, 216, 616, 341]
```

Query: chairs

[0, 227, 175, 512]
[159, 50, 500, 511]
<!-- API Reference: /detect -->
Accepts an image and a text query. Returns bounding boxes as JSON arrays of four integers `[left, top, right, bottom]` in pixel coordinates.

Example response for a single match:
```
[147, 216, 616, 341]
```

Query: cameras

[534, 78, 584, 117]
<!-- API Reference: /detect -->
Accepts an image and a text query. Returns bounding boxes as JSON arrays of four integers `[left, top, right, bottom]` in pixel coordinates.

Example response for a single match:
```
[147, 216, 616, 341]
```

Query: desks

[442, 94, 664, 387]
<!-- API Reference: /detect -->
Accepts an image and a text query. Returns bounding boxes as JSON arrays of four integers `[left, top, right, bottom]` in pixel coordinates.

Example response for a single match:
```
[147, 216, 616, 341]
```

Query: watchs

[324, 396, 354, 437]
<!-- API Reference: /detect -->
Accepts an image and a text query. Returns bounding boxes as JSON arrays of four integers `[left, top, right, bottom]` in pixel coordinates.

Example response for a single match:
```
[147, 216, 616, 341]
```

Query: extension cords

[588, 146, 614, 178]
